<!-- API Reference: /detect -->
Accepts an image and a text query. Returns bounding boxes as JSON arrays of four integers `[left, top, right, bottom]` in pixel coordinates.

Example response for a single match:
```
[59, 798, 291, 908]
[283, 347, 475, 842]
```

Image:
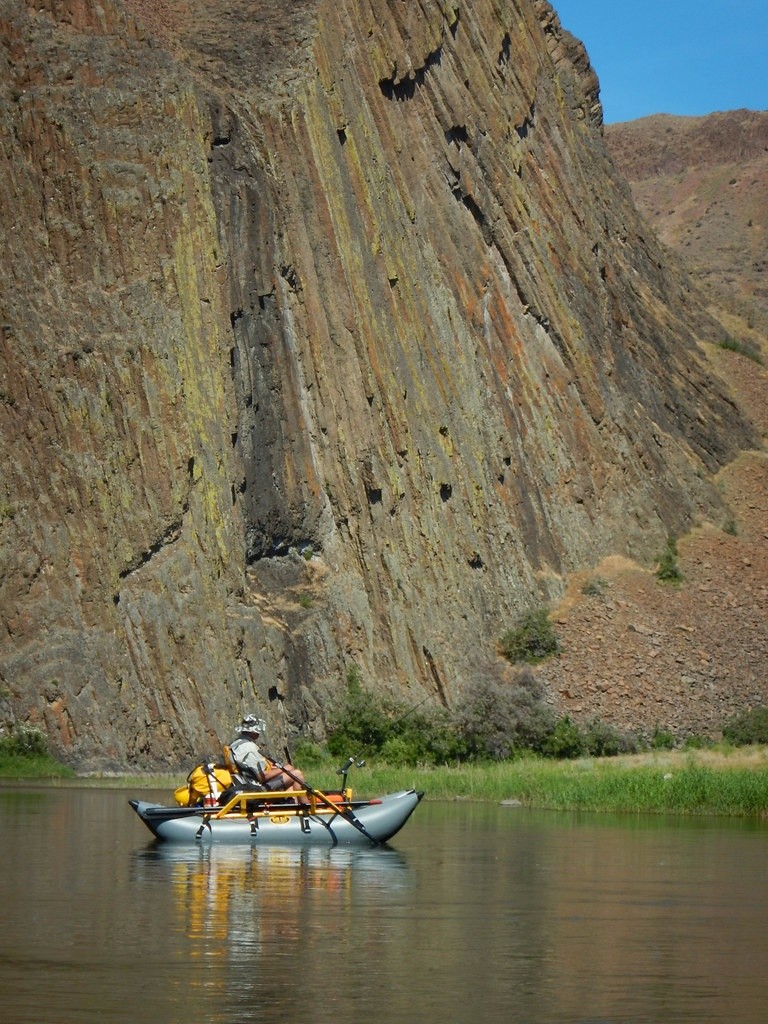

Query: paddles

[258, 748, 394, 849]
[283, 745, 294, 767]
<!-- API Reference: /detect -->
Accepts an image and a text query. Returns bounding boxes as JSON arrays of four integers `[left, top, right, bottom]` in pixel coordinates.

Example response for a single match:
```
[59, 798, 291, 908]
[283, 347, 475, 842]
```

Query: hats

[235, 714, 266, 733]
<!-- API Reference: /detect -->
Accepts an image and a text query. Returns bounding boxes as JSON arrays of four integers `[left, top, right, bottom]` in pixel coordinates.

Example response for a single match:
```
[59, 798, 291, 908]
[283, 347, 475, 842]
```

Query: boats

[128, 788, 425, 842]
[139, 842, 411, 886]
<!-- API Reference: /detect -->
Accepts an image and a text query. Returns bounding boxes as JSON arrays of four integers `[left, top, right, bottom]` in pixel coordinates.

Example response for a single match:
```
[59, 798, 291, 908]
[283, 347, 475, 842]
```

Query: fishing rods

[335, 664, 470, 776]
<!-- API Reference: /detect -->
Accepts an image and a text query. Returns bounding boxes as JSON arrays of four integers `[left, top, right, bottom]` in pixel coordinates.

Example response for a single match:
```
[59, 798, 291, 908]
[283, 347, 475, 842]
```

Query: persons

[229, 714, 310, 804]
